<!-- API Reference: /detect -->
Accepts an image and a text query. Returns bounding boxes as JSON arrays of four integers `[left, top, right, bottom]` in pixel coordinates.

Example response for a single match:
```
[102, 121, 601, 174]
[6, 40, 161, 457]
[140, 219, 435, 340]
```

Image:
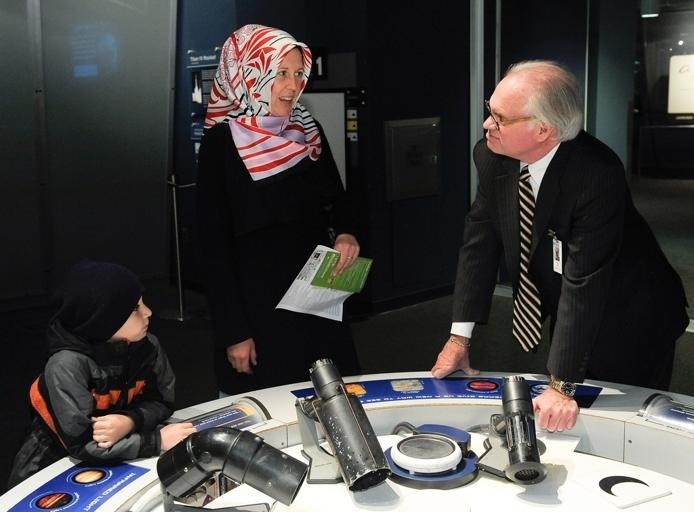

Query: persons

[431, 59, 690, 434]
[7, 263, 198, 490]
[193, 23, 362, 398]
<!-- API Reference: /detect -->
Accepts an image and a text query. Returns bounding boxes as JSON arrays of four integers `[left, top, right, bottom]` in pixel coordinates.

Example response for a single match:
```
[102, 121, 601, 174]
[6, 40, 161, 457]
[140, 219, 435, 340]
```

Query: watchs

[550, 374, 577, 398]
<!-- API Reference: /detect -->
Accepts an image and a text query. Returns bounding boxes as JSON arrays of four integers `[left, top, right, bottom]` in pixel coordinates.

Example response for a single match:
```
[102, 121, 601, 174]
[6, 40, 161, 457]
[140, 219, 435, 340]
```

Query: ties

[512, 166, 543, 352]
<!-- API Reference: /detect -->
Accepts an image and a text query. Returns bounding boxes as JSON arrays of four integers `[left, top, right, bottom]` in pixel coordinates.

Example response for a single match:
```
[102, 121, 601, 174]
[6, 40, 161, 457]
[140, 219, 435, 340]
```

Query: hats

[57, 262, 147, 343]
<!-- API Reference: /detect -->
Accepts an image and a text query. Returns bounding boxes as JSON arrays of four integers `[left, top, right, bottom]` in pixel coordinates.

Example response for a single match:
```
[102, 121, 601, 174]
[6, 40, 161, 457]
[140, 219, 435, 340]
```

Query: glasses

[485, 100, 534, 129]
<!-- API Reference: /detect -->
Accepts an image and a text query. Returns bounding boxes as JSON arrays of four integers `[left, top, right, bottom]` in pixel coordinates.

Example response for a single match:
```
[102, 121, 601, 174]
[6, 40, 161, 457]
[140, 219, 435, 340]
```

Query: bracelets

[449, 337, 471, 348]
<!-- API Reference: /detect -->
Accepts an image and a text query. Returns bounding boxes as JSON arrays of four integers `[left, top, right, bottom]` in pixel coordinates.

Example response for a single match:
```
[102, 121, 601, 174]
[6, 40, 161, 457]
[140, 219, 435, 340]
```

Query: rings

[348, 256, 353, 259]
